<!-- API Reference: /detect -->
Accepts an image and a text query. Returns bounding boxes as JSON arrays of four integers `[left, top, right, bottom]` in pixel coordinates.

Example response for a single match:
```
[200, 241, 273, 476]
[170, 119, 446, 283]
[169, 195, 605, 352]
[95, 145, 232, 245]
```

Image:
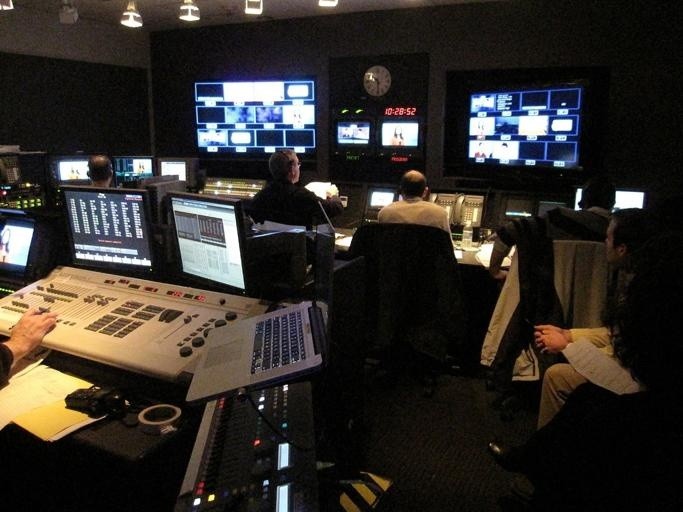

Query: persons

[490, 176, 682, 511]
[392, 126, 405, 145]
[0, 228, 11, 263]
[377, 169, 454, 248]
[86, 154, 114, 187]
[0, 308, 58, 389]
[248, 149, 343, 232]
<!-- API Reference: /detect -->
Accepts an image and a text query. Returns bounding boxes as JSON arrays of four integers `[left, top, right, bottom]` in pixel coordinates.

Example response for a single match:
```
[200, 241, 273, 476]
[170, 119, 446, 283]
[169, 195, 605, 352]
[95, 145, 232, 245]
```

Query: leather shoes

[486, 437, 528, 476]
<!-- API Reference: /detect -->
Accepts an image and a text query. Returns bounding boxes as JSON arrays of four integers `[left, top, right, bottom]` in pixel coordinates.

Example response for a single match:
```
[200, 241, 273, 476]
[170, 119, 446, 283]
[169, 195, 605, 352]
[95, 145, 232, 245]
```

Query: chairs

[351, 223, 461, 398]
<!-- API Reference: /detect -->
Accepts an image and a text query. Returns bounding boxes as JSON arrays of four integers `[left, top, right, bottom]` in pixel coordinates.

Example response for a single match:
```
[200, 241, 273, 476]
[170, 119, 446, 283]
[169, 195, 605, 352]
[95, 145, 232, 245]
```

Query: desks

[1, 336, 194, 463]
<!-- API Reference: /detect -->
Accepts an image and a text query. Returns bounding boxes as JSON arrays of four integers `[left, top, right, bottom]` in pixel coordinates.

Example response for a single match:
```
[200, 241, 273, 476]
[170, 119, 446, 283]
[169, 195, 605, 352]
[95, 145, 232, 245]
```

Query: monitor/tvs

[194, 80, 317, 156]
[573, 186, 646, 214]
[167, 190, 249, 292]
[157, 158, 188, 182]
[112, 155, 156, 186]
[59, 185, 154, 269]
[370, 191, 394, 207]
[381, 122, 420, 148]
[467, 87, 583, 171]
[56, 155, 90, 183]
[0, 218, 36, 274]
[337, 121, 370, 145]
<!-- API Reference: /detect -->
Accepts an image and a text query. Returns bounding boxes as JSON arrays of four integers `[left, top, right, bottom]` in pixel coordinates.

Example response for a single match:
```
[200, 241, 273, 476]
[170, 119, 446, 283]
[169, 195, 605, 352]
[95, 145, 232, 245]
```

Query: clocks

[362, 64, 391, 96]
